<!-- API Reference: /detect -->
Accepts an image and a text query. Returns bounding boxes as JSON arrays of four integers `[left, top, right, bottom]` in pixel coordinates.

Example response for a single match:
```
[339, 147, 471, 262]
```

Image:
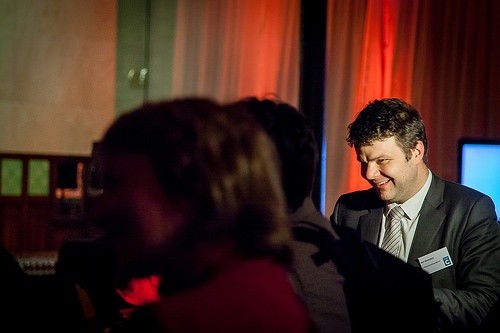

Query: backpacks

[290, 221, 443, 333]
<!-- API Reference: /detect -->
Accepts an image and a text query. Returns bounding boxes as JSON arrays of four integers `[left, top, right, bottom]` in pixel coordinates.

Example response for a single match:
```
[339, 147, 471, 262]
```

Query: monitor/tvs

[457, 137, 498, 219]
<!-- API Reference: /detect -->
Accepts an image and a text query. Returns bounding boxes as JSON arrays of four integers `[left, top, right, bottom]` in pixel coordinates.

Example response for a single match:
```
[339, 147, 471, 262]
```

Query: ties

[381, 206, 404, 258]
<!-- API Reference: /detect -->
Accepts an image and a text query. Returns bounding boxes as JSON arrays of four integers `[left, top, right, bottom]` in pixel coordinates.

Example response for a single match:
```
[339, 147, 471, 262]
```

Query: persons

[209, 93, 438, 333]
[86, 97, 314, 333]
[327, 97, 499, 333]
